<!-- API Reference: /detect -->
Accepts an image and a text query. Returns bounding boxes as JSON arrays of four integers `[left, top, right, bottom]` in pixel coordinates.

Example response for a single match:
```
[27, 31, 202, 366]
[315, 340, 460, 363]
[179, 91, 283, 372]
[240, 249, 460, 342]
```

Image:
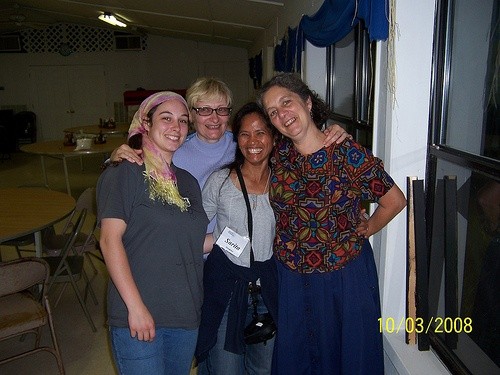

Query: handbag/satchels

[244, 315, 277, 344]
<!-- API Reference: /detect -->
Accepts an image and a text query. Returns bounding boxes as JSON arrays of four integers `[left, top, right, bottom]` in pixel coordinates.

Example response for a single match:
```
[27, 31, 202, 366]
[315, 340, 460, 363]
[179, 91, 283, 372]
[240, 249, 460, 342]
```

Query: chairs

[0, 187, 101, 375]
[0, 109, 37, 162]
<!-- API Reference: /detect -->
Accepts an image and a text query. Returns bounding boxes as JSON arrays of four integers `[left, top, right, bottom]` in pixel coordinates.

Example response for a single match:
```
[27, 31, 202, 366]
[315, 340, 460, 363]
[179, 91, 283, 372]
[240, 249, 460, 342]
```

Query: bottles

[99, 118, 116, 129]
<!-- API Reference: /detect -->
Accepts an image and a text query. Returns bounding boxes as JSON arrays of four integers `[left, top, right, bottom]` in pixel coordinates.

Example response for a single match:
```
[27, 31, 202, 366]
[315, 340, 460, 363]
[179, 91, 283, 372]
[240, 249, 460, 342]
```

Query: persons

[258, 72, 409, 375]
[94, 91, 215, 375]
[197, 103, 275, 375]
[109, 76, 353, 262]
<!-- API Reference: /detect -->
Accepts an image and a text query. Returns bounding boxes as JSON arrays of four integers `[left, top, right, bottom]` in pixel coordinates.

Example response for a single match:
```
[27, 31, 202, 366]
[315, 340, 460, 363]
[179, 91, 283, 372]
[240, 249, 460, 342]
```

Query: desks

[64, 122, 130, 173]
[0, 188, 77, 295]
[20, 137, 127, 199]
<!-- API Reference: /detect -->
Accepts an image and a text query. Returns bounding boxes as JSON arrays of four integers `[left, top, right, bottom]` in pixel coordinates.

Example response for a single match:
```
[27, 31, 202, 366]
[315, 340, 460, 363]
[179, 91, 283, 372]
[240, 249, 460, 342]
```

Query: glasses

[192, 107, 232, 117]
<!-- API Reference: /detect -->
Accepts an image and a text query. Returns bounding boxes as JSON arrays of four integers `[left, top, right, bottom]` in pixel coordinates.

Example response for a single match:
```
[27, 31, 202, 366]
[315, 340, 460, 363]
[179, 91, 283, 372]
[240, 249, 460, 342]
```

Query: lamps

[98, 11, 127, 28]
